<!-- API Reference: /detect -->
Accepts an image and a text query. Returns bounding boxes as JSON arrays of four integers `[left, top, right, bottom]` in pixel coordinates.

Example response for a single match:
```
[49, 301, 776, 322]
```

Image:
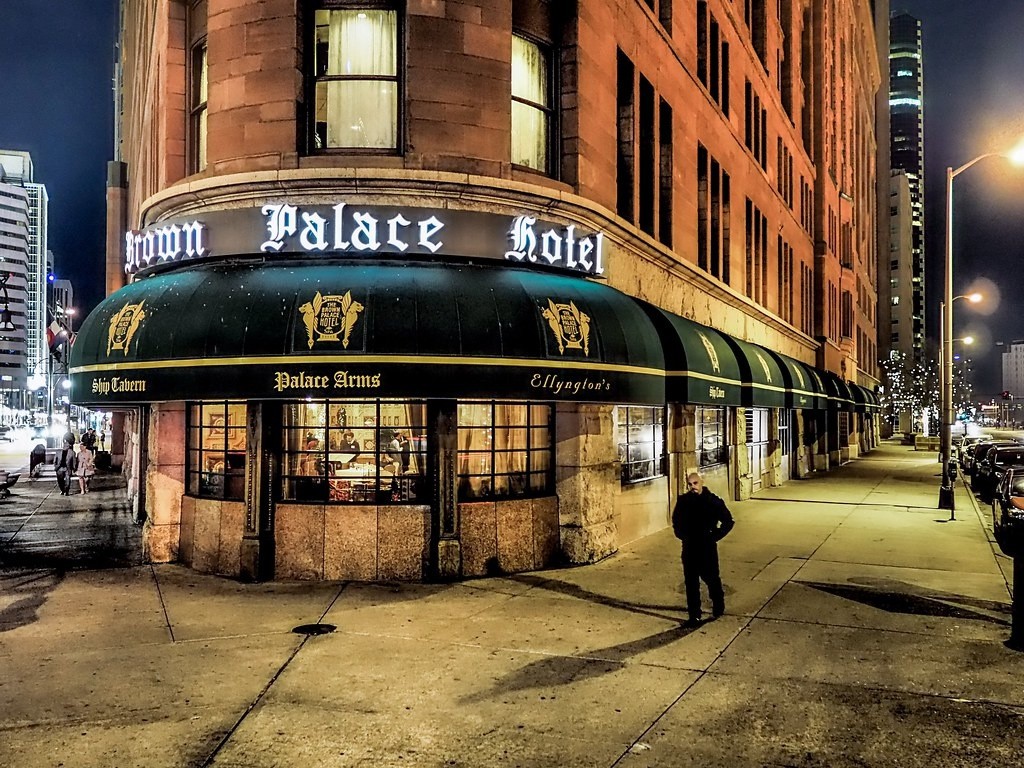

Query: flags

[46, 307, 69, 363]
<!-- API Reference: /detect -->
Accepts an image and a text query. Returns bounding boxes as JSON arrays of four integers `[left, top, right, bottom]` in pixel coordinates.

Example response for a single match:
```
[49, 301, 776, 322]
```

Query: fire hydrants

[0, 470, 21, 499]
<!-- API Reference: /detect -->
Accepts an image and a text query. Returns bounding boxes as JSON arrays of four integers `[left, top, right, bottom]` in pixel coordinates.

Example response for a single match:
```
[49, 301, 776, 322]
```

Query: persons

[380, 429, 410, 477]
[53, 428, 105, 497]
[670, 472, 733, 628]
[340, 429, 361, 463]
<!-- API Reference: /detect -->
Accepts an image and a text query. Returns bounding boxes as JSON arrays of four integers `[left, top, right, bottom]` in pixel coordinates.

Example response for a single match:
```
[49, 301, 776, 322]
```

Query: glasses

[688, 481, 698, 485]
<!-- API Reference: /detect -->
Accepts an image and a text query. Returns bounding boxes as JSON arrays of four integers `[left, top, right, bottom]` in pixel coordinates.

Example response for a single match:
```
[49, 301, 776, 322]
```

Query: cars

[951, 434, 1024, 552]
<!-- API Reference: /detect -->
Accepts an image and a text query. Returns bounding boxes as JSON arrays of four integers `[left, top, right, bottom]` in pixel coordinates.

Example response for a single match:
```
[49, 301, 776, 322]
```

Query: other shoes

[712, 592, 725, 618]
[66, 491, 69, 496]
[85, 487, 90, 492]
[60, 491, 64, 495]
[81, 491, 85, 495]
[682, 617, 702, 631]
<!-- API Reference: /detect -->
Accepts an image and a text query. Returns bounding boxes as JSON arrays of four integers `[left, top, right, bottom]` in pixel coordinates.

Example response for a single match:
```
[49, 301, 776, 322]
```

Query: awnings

[69, 254, 665, 407]
[650, 302, 884, 415]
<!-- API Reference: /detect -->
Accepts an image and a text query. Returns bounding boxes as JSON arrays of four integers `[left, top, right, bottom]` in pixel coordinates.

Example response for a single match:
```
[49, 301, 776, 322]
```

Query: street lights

[938, 293, 983, 462]
[936, 136, 1024, 511]
